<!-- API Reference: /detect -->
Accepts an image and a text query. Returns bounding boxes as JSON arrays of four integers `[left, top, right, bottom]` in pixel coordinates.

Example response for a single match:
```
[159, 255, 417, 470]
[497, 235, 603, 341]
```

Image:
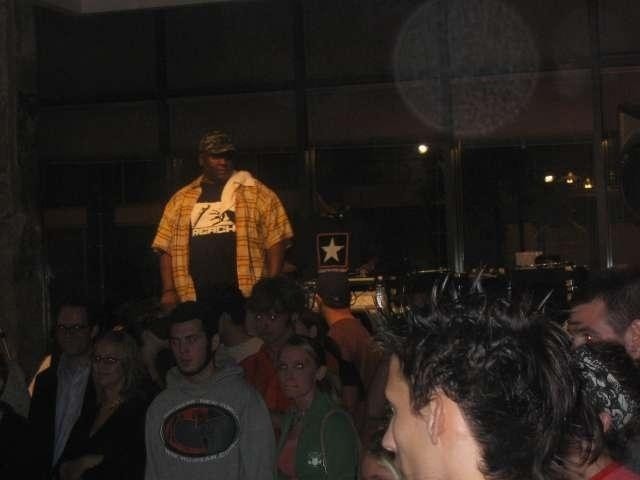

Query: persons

[1, 266, 639, 480]
[150, 131, 296, 308]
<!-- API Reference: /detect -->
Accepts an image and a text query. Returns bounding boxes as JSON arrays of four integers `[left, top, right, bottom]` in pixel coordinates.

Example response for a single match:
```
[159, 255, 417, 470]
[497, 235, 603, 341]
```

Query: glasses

[58, 323, 85, 332]
[92, 355, 123, 365]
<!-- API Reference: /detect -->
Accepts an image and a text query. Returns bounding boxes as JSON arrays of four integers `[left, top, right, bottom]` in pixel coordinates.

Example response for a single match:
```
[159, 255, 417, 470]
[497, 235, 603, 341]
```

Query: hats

[199, 129, 237, 156]
[318, 271, 350, 307]
